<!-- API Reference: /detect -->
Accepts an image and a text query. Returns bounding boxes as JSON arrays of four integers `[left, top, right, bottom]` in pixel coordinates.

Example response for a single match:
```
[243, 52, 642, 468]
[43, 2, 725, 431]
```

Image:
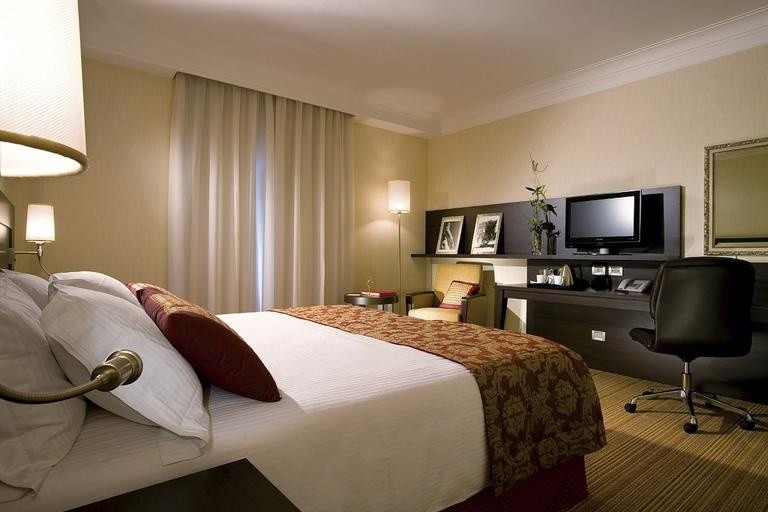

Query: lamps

[0, 0, 90, 180]
[13, 204, 57, 276]
[387, 178, 413, 314]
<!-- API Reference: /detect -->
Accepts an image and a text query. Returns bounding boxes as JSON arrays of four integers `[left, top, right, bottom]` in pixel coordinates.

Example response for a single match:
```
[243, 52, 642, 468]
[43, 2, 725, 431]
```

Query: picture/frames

[469, 211, 504, 255]
[434, 214, 465, 254]
[702, 136, 768, 257]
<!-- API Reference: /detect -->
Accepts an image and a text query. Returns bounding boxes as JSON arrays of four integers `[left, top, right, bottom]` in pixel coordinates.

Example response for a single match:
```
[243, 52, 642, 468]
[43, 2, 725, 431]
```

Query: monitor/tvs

[565, 190, 642, 255]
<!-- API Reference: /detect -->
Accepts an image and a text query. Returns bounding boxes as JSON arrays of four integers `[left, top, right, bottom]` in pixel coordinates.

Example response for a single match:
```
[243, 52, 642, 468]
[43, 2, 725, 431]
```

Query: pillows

[437, 279, 481, 310]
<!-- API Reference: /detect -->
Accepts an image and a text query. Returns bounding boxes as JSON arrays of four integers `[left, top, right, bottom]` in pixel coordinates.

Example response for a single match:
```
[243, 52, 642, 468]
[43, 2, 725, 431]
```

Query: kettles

[558, 263, 574, 288]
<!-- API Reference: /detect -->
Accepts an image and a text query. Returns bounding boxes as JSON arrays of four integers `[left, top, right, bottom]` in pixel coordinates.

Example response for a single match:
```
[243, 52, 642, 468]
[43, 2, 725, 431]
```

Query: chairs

[405, 261, 495, 328]
[625, 256, 756, 434]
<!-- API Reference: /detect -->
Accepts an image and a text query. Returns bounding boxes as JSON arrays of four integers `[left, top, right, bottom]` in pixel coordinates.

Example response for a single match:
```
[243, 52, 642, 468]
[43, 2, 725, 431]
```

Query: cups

[539, 269, 553, 276]
[536, 274, 563, 285]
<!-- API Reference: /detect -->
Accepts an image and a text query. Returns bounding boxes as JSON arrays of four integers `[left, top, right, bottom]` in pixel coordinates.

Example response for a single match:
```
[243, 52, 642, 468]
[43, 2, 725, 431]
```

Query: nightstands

[62, 455, 299, 511]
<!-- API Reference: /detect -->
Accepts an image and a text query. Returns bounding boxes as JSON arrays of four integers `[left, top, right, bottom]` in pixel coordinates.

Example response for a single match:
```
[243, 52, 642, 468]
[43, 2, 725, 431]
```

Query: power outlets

[608, 264, 624, 277]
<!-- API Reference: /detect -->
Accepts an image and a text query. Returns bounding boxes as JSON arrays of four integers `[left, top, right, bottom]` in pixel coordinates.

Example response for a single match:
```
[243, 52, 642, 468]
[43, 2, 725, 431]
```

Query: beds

[1, 190, 610, 512]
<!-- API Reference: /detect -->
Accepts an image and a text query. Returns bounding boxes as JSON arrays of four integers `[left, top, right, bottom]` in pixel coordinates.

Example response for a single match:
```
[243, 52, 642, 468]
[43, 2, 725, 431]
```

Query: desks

[343, 291, 399, 313]
[493, 253, 768, 408]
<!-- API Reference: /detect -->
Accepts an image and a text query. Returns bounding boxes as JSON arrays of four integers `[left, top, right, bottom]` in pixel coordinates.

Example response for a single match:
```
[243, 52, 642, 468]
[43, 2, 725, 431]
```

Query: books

[359, 289, 397, 296]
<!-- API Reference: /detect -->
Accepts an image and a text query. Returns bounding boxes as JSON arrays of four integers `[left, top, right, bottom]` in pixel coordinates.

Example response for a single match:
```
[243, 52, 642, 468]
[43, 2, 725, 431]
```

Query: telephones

[616, 278, 652, 293]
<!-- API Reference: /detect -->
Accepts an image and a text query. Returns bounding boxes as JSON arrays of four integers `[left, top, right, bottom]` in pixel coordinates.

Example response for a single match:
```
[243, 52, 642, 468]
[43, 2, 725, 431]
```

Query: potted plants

[523, 149, 561, 255]
